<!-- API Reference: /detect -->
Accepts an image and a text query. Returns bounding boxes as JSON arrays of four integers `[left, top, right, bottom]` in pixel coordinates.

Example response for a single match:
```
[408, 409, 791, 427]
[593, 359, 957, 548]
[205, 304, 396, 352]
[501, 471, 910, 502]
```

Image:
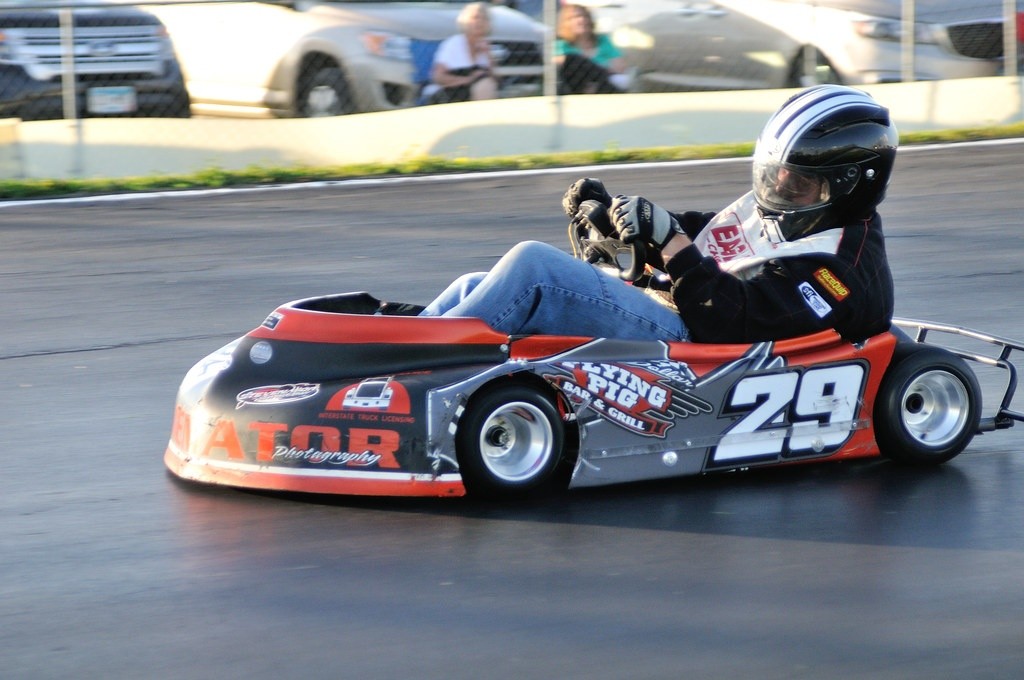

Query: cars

[710, 0, 1024, 86]
[134, 0, 566, 118]
[504, 0, 845, 93]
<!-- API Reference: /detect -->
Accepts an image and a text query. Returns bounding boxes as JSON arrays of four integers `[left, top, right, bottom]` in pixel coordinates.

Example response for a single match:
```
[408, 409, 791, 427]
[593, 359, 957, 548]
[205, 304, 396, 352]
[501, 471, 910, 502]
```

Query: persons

[375, 84, 900, 343]
[552, 4, 629, 94]
[417, 1, 499, 100]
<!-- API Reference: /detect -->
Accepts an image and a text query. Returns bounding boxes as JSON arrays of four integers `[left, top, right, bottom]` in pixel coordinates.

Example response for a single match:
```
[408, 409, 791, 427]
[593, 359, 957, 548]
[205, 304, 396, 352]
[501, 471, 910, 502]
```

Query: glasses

[763, 160, 817, 196]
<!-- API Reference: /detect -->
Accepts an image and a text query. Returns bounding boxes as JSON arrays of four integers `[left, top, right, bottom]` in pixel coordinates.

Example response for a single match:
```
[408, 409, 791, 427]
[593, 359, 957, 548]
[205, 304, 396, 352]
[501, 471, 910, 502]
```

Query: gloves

[561, 177, 612, 218]
[610, 196, 685, 251]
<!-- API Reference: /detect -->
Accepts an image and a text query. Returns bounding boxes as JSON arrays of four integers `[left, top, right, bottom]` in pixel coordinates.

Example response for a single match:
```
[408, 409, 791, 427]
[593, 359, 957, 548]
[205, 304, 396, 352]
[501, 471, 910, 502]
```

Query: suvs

[0, 0, 191, 121]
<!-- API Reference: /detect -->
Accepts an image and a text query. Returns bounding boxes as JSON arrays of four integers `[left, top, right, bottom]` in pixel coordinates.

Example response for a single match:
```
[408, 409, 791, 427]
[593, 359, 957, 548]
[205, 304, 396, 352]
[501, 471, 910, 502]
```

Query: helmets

[752, 84, 900, 244]
